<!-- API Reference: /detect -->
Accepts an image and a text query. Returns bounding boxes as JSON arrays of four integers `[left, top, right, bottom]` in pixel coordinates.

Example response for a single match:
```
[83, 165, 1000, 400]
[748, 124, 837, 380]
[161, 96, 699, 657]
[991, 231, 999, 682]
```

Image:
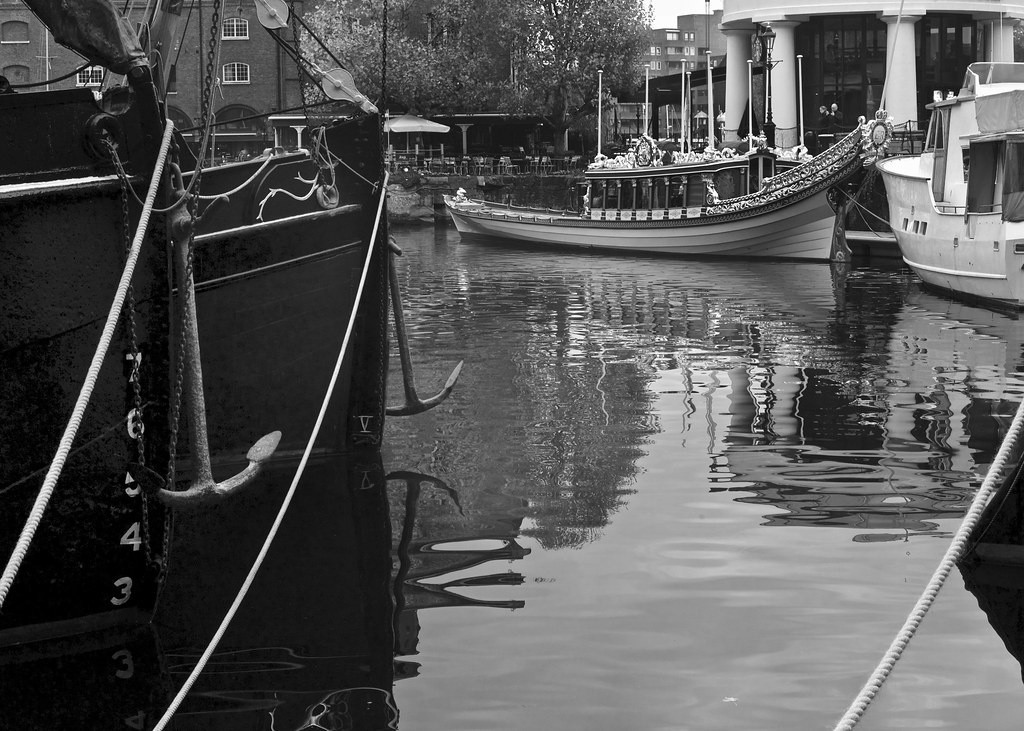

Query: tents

[383, 113, 450, 152]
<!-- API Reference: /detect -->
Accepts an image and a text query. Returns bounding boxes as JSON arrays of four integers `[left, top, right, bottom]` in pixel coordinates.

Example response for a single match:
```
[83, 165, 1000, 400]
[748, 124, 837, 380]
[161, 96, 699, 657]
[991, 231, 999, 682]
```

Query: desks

[551, 159, 576, 170]
[512, 159, 523, 172]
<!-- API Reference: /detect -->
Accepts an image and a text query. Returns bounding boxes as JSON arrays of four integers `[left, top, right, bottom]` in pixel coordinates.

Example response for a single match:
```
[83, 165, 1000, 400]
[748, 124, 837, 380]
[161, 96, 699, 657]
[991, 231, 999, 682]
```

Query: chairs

[385, 155, 578, 176]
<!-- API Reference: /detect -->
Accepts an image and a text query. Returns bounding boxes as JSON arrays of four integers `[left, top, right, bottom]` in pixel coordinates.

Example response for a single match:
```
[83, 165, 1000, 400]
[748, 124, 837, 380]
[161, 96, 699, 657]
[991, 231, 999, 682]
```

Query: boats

[442, 49, 894, 266]
[0, 0, 403, 731]
[874, 61, 1024, 317]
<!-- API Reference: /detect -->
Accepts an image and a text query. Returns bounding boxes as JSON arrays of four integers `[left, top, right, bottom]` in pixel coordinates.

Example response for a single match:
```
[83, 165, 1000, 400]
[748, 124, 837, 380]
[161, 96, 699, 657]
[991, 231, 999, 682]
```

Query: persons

[819, 103, 843, 129]
[511, 145, 529, 175]
